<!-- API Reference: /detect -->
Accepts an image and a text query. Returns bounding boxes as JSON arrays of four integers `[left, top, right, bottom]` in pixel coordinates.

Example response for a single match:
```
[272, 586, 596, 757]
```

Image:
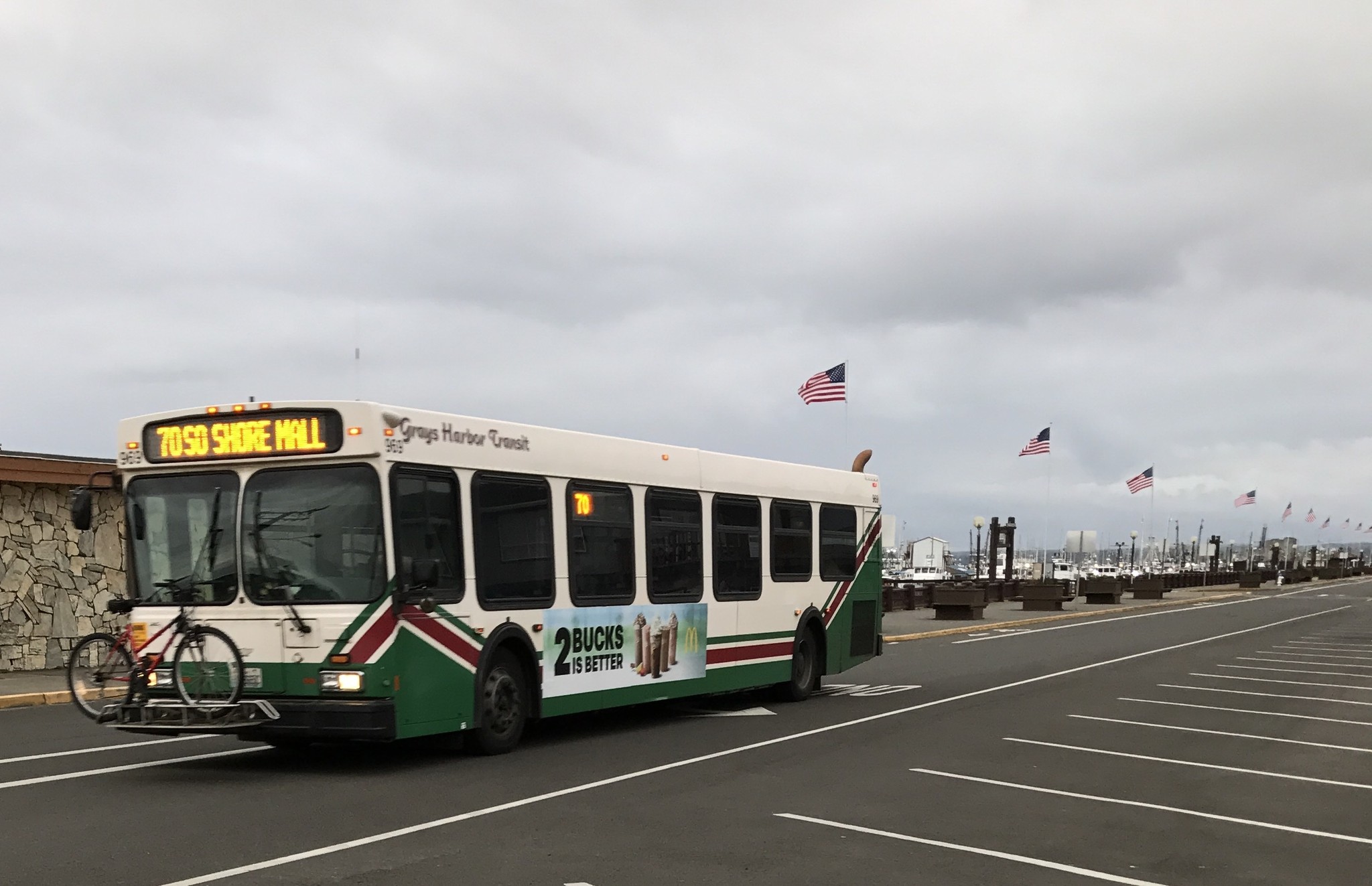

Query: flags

[1235, 489, 1257, 508]
[1280, 501, 1372, 536]
[1125, 465, 1155, 494]
[1018, 427, 1052, 457]
[798, 361, 848, 405]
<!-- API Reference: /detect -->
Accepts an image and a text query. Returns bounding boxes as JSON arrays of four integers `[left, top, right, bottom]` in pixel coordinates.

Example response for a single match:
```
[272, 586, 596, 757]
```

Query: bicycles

[66, 576, 247, 725]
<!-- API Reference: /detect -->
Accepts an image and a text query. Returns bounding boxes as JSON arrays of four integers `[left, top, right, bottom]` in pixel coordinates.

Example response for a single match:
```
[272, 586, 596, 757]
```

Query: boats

[882, 512, 1271, 590]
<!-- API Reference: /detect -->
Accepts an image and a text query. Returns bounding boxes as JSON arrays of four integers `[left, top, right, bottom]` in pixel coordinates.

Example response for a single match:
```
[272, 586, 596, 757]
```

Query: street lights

[972, 514, 985, 584]
[1228, 539, 1235, 571]
[1189, 535, 1199, 571]
[1128, 530, 1139, 573]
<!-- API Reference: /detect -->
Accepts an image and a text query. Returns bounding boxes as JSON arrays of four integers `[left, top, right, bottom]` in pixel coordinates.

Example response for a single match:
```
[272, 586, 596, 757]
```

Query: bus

[68, 400, 886, 757]
